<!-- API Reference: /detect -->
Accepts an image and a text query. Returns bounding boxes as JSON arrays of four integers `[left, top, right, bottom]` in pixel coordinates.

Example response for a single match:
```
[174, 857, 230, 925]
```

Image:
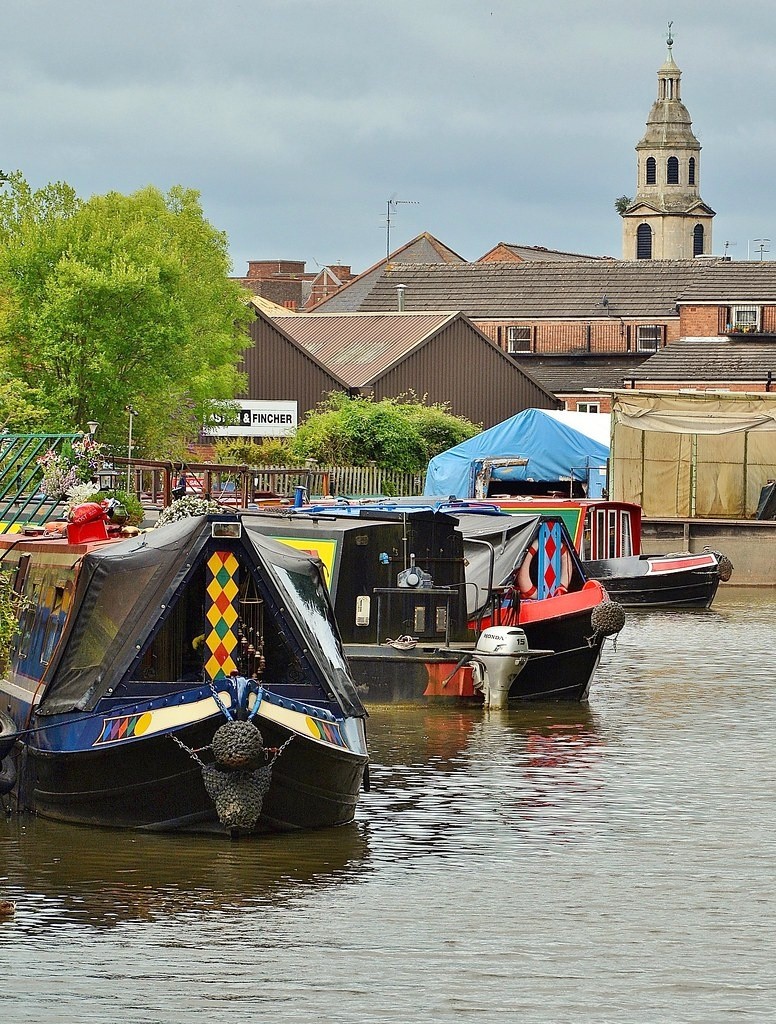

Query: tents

[422, 407, 610, 498]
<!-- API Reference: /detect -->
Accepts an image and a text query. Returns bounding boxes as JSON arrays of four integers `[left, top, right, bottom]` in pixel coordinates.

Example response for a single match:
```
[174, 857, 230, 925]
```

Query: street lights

[87, 419, 100, 445]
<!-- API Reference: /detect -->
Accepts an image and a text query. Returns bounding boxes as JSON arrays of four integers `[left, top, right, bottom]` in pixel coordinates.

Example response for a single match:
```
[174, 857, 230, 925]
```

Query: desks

[373, 588, 460, 647]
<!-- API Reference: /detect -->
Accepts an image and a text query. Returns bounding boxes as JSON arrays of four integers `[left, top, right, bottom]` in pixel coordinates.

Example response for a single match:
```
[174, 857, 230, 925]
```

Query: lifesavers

[517, 537, 574, 600]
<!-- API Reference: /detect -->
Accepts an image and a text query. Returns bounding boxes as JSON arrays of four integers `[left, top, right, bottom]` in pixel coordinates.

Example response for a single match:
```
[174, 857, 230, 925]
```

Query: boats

[213, 485, 627, 711]
[0, 496, 372, 842]
[310, 497, 732, 609]
